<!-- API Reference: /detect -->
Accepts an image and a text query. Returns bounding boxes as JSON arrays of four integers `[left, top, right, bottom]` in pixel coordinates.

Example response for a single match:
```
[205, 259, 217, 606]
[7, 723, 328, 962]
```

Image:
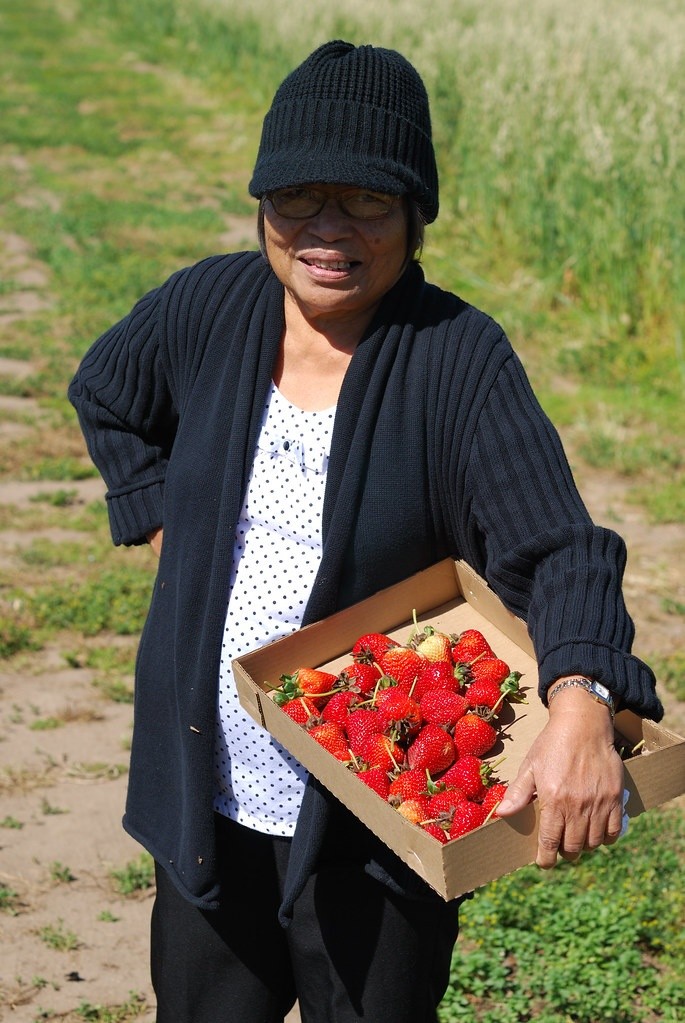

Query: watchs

[548, 678, 616, 726]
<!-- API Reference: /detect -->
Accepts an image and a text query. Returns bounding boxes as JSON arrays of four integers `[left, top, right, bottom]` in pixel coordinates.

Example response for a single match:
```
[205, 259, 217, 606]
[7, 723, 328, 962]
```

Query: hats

[248, 39, 440, 221]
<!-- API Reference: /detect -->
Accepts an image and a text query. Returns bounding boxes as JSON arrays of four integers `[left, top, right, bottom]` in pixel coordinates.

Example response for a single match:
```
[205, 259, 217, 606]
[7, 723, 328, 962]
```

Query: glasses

[260, 185, 401, 220]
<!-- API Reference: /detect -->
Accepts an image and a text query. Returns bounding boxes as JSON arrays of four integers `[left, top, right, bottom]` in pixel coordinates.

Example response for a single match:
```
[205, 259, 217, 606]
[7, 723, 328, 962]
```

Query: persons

[68, 42, 667, 1022]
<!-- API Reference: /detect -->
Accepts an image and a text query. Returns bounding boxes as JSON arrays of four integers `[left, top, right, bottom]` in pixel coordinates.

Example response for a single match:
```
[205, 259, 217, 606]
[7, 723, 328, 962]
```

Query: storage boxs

[230, 554, 683, 904]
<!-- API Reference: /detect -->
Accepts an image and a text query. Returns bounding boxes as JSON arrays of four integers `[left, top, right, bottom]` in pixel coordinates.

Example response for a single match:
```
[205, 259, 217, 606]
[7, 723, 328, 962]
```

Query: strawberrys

[273, 630, 513, 847]
